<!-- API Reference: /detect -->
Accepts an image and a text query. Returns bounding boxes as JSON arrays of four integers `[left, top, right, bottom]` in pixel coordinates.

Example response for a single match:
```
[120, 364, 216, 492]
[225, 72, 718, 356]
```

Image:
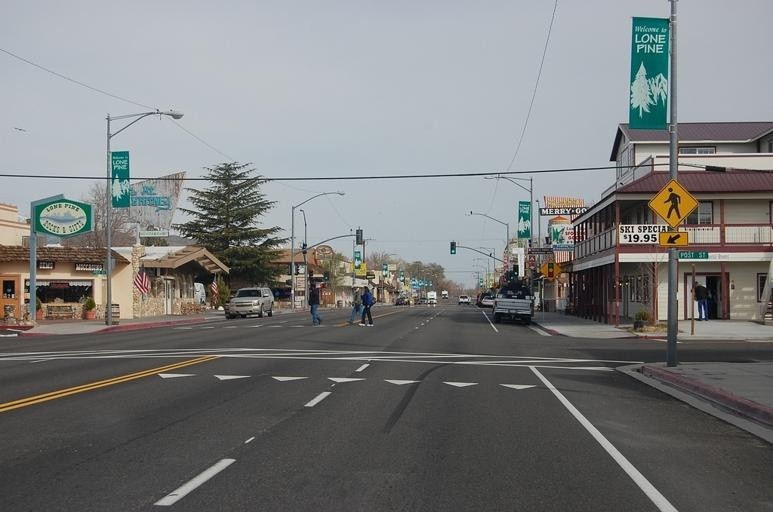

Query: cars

[481, 296, 493, 306]
[418, 298, 437, 304]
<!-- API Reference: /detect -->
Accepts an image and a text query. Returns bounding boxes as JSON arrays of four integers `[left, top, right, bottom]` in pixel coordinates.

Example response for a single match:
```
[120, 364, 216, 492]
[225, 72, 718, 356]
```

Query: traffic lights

[451, 242, 456, 254]
[540, 261, 561, 281]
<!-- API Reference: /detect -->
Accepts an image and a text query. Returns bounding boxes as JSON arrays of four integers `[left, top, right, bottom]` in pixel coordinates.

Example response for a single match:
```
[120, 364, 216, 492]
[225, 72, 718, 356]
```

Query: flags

[135, 265, 152, 295]
[210, 276, 220, 304]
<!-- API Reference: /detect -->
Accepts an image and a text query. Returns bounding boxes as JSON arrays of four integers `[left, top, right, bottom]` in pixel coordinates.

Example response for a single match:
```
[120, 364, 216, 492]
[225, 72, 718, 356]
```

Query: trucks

[442, 290, 448, 299]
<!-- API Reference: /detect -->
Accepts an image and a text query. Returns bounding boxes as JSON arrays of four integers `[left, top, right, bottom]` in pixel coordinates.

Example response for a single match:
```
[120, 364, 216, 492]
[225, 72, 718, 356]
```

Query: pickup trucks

[493, 288, 533, 325]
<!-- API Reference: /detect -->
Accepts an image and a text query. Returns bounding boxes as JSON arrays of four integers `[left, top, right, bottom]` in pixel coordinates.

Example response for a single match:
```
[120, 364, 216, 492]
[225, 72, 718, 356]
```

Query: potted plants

[36, 297, 42, 319]
[634, 311, 650, 329]
[84, 299, 97, 319]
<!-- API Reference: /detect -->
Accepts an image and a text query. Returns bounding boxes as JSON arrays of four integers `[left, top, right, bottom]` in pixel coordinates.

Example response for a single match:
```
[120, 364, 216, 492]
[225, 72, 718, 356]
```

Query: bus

[428, 291, 436, 299]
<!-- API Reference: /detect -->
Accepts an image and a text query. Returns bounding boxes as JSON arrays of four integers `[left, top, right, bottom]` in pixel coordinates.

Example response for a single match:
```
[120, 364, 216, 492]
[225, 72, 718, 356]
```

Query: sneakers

[359, 323, 374, 327]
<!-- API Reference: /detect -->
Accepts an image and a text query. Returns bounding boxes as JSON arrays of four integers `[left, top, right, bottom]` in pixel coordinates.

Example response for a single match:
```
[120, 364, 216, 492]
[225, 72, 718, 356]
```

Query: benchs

[46, 305, 73, 319]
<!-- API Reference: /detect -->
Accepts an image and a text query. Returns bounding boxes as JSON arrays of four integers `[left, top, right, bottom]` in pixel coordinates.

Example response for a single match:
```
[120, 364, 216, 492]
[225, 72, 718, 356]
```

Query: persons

[306, 286, 321, 326]
[691, 279, 710, 323]
[346, 285, 363, 326]
[361, 286, 377, 328]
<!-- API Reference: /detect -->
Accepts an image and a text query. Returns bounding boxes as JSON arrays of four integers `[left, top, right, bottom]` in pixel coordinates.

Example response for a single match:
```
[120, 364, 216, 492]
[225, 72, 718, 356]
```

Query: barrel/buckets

[105, 304, 119, 326]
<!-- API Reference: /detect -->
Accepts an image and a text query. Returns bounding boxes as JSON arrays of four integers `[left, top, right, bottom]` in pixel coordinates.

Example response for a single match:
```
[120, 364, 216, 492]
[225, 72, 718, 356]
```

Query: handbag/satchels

[371, 297, 377, 304]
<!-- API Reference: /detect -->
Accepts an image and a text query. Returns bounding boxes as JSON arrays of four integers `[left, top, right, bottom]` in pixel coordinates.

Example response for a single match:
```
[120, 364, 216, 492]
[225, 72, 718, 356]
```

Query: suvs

[459, 295, 470, 305]
[230, 287, 275, 319]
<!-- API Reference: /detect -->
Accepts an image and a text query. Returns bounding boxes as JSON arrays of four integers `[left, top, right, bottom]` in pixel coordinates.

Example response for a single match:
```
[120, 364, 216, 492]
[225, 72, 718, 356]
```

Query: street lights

[466, 176, 540, 297]
[291, 192, 345, 310]
[106, 109, 184, 325]
